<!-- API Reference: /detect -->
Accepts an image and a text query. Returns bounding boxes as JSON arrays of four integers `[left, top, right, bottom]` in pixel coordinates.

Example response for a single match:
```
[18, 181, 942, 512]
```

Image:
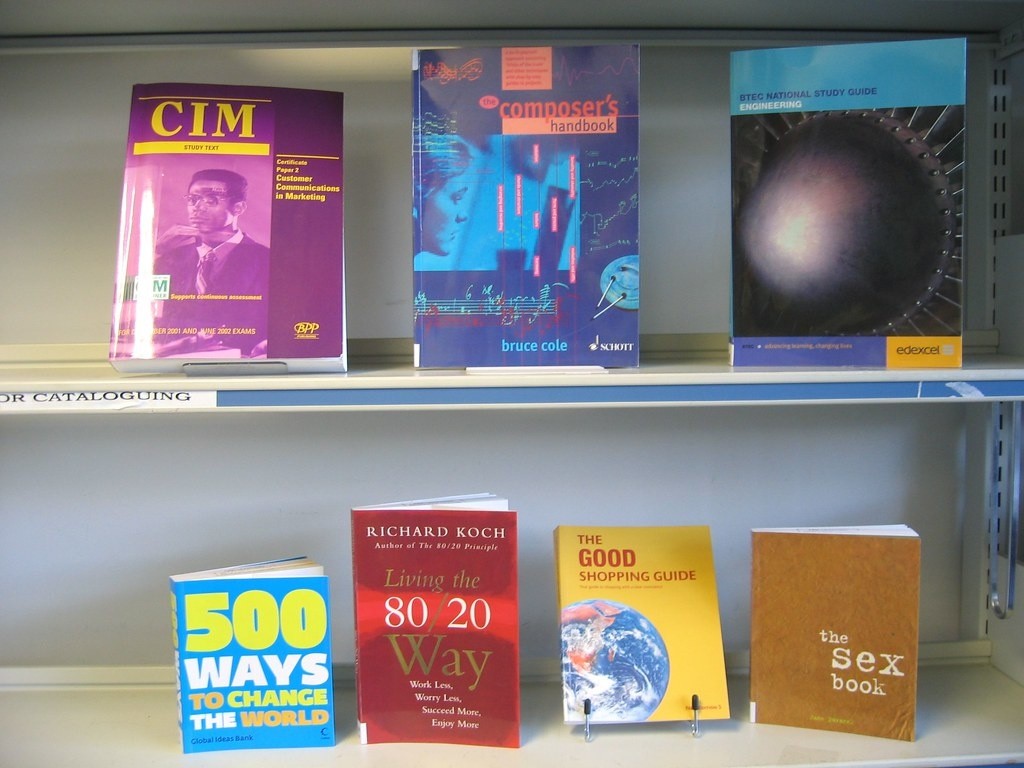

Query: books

[727, 36, 966, 368]
[110, 82, 348, 375]
[412, 43, 641, 370]
[750, 524, 919, 743]
[169, 555, 337, 753]
[554, 524, 730, 724]
[353, 492, 521, 749]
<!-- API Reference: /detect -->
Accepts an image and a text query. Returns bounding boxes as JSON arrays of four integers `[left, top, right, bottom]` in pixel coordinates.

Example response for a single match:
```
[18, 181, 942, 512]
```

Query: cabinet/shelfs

[0, 0, 1024, 768]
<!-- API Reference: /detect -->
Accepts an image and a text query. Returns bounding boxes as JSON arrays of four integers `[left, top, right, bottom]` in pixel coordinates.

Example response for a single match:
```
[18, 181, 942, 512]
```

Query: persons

[149, 167, 269, 356]
[413, 134, 472, 260]
